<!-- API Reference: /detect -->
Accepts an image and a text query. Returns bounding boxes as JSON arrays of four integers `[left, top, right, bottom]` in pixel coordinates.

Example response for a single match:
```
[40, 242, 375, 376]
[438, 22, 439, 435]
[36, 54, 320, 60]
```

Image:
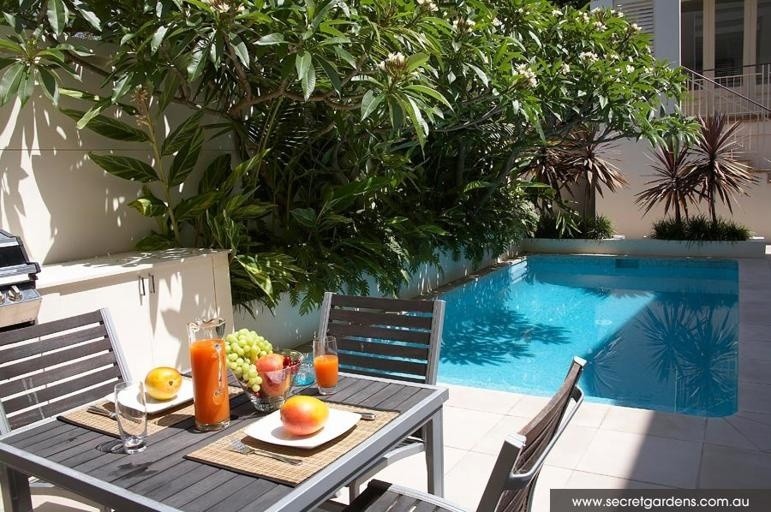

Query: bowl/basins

[228, 348, 304, 412]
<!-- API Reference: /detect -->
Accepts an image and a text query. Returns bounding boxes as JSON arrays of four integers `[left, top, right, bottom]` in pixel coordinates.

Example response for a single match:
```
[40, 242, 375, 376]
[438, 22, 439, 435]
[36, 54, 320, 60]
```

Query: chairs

[1, 290, 589, 512]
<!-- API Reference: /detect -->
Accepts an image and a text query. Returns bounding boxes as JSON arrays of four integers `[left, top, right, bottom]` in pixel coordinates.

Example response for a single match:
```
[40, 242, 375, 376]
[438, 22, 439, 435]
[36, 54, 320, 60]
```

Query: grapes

[224, 330, 273, 392]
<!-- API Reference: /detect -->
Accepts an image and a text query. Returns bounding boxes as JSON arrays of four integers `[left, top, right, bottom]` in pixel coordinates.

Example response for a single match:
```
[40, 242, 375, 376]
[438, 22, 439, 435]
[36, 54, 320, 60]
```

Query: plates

[104, 377, 193, 416]
[243, 405, 362, 450]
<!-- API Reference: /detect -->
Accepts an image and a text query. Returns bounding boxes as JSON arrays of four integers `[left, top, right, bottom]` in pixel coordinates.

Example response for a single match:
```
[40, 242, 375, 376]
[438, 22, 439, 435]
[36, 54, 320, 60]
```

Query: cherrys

[283, 356, 300, 371]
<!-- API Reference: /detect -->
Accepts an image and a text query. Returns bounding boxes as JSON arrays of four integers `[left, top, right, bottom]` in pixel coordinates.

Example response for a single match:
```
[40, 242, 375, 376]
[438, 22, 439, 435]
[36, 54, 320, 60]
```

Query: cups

[113, 380, 149, 455]
[291, 352, 314, 386]
[313, 336, 340, 396]
[185, 317, 231, 433]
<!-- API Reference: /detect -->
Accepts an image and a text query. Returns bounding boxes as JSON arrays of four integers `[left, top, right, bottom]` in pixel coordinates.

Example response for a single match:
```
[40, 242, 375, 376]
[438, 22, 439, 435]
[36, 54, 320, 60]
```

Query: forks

[229, 439, 304, 467]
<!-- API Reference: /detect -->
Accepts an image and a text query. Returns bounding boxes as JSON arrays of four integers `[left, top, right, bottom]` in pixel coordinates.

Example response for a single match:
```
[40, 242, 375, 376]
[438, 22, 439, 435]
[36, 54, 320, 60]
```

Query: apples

[145, 367, 182, 400]
[280, 394, 328, 436]
[257, 354, 290, 397]
